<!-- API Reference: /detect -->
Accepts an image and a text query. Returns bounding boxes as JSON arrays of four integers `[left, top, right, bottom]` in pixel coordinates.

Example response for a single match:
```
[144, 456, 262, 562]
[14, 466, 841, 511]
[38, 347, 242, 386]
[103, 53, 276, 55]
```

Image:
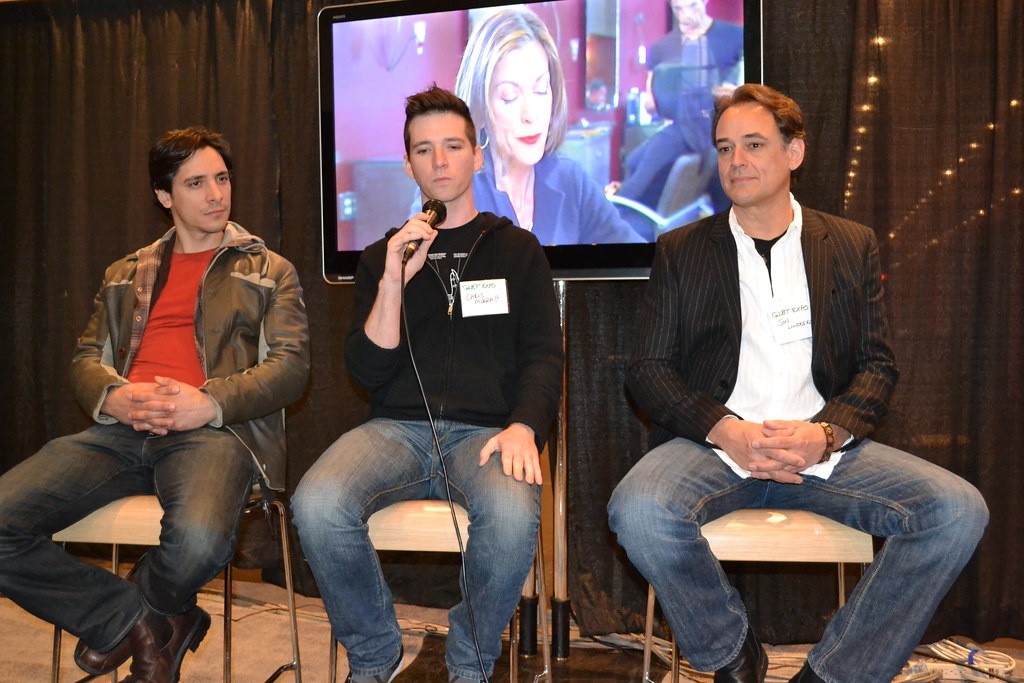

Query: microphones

[401, 198, 448, 264]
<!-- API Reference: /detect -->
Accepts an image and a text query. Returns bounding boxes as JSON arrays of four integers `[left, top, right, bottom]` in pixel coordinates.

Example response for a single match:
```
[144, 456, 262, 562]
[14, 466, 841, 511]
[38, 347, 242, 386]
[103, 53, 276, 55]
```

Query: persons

[607, 83, 989, 683]
[409, 7, 649, 245]
[1, 126, 314, 683]
[289, 87, 562, 683]
[606, 0, 745, 222]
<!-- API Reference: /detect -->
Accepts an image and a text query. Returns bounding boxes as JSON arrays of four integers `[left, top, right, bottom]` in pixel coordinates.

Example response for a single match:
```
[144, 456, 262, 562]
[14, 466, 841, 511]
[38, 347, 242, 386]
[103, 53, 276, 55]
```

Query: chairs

[46, 277, 874, 683]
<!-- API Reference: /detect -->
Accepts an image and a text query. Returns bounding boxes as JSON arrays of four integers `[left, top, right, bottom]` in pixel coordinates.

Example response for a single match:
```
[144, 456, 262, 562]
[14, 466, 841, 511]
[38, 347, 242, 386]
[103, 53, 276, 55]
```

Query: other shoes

[344, 644, 404, 683]
[448, 670, 486, 683]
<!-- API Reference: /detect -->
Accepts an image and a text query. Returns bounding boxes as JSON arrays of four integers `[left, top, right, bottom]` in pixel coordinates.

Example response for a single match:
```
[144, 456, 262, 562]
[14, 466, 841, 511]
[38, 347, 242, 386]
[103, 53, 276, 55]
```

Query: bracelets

[819, 421, 834, 464]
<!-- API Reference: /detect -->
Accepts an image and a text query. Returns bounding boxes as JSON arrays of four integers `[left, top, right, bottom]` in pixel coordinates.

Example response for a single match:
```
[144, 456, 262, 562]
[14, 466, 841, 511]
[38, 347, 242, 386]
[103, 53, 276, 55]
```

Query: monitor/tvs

[318, 0, 767, 285]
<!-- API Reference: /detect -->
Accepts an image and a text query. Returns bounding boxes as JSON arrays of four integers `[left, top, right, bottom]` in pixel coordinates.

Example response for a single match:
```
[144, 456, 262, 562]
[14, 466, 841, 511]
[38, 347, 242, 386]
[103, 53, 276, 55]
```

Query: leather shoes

[74, 550, 149, 676]
[119, 605, 211, 683]
[789, 660, 825, 683]
[714, 624, 769, 683]
